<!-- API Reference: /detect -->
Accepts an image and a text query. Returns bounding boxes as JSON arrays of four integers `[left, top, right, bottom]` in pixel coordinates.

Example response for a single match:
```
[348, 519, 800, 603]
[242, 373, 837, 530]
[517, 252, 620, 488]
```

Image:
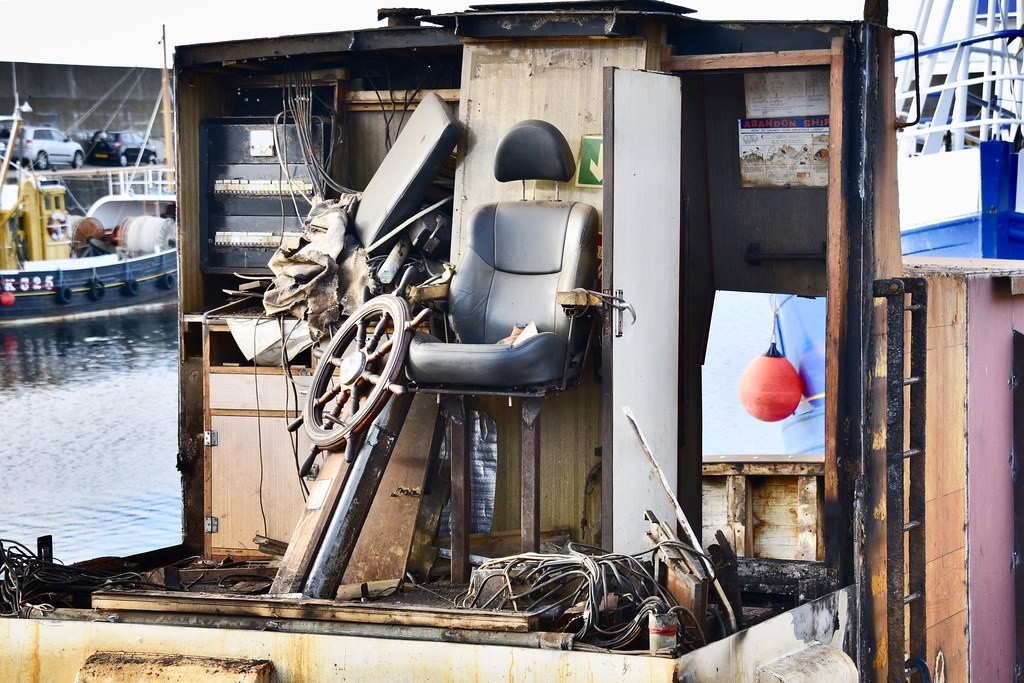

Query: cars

[0, 126, 85, 169]
[86, 129, 160, 165]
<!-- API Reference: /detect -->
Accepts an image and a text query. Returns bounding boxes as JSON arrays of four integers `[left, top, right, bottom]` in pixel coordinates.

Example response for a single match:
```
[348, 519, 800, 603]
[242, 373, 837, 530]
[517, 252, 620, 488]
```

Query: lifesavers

[47, 213, 66, 240]
[59, 287, 72, 303]
[91, 280, 105, 300]
[126, 280, 139, 296]
[163, 275, 174, 289]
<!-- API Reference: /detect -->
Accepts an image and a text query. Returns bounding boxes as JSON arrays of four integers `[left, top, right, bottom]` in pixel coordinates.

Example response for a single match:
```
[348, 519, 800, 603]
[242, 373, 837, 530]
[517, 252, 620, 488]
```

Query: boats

[1, 169, 177, 328]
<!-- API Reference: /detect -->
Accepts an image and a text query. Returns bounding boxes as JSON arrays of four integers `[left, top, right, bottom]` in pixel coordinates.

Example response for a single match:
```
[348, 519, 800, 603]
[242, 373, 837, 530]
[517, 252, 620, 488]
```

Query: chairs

[400, 119, 604, 396]
